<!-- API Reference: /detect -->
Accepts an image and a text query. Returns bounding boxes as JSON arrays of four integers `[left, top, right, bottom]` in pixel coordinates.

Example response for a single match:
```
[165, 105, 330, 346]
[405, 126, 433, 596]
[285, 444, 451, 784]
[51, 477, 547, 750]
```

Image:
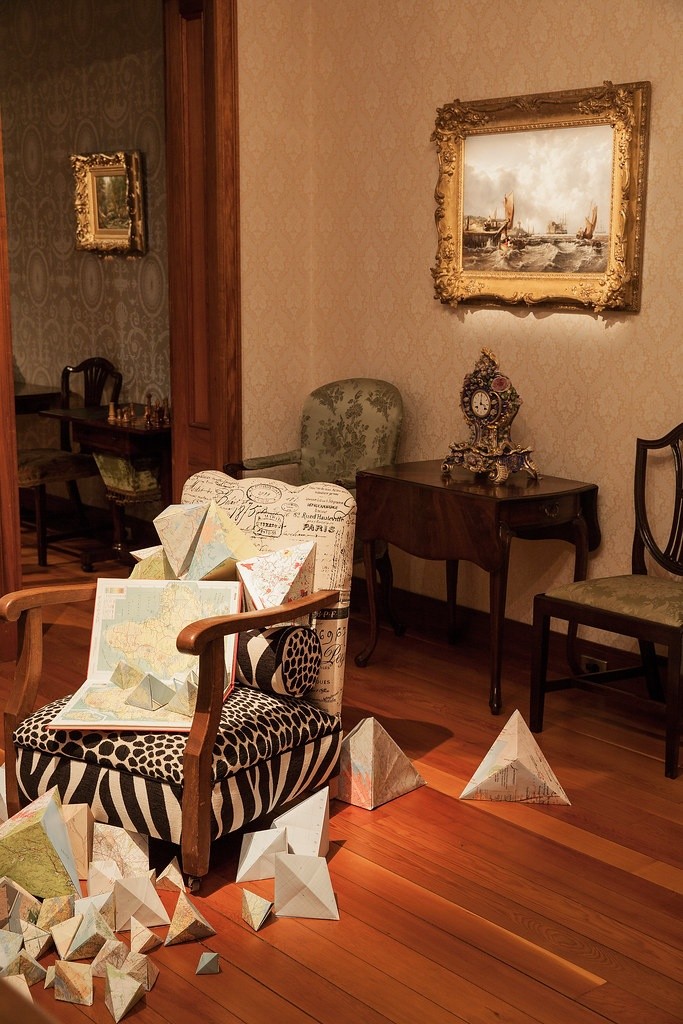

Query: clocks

[441, 346, 545, 483]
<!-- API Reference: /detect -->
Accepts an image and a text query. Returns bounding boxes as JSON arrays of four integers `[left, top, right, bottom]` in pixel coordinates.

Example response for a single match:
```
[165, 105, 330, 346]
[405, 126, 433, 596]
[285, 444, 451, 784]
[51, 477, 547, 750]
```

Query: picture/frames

[428, 79, 652, 314]
[69, 149, 147, 257]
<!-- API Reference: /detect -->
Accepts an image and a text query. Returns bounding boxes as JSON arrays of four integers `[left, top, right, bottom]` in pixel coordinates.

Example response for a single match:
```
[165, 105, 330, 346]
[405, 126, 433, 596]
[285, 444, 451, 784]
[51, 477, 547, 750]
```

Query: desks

[40, 404, 171, 558]
[15, 383, 61, 414]
[351, 457, 603, 716]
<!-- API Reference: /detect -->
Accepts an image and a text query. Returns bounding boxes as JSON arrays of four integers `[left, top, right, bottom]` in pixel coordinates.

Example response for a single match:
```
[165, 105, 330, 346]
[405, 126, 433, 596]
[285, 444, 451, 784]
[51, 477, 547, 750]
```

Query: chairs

[0, 466, 357, 890]
[97, 449, 170, 556]
[223, 378, 406, 671]
[17, 357, 124, 565]
[529, 424, 683, 782]
